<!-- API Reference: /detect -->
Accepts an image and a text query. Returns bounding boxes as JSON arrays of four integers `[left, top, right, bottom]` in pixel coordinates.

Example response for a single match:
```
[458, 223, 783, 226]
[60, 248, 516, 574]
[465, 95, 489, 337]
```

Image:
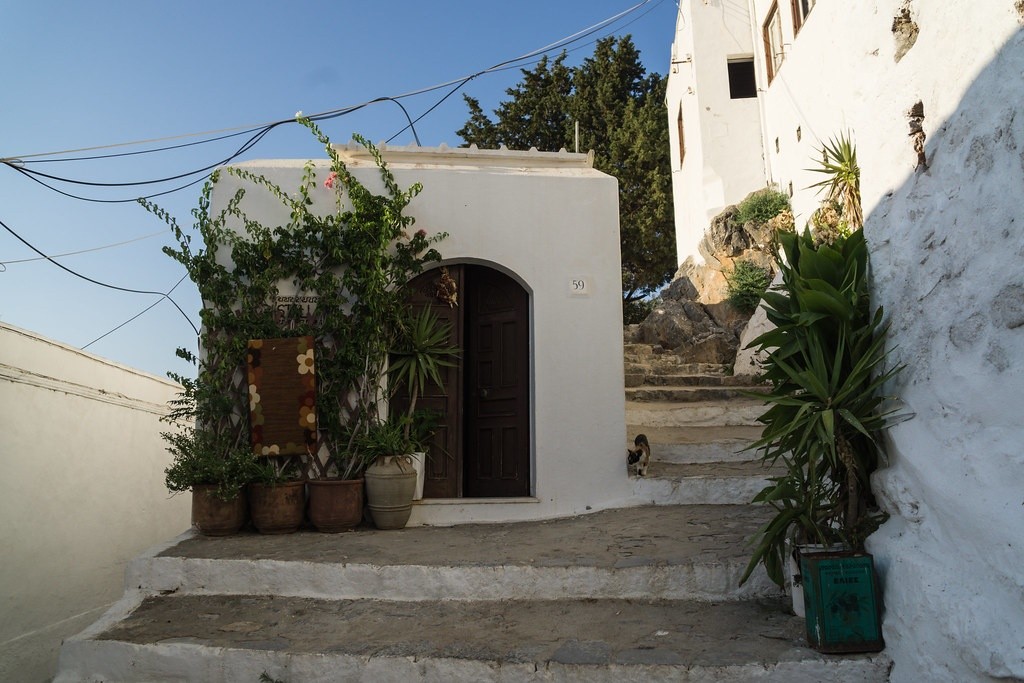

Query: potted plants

[732, 224, 914, 654]
[138, 111, 464, 537]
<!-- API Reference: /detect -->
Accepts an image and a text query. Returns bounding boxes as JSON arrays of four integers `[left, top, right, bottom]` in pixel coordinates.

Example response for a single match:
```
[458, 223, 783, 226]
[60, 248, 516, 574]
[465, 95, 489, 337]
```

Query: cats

[627, 434, 650, 478]
